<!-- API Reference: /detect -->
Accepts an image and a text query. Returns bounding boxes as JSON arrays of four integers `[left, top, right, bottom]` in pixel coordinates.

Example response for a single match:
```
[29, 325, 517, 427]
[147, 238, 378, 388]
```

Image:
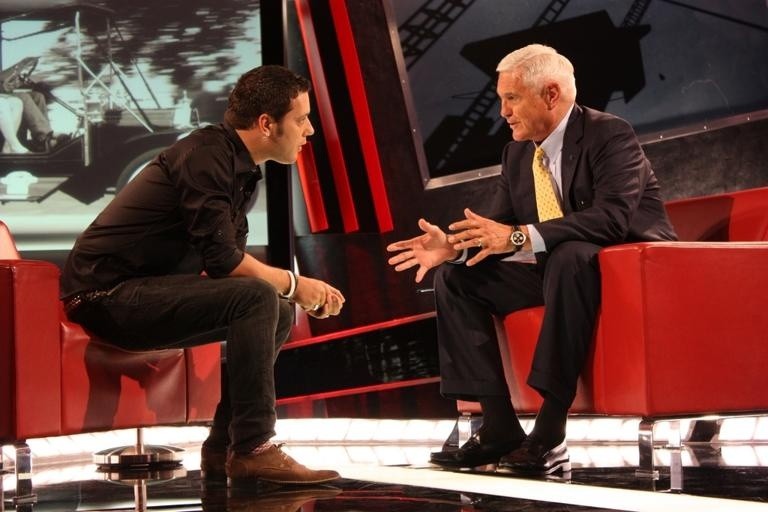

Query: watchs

[510, 225, 527, 253]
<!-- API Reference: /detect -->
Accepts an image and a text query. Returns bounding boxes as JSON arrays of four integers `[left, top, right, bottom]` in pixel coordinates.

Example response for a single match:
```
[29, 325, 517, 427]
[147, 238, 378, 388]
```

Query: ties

[533, 147, 565, 224]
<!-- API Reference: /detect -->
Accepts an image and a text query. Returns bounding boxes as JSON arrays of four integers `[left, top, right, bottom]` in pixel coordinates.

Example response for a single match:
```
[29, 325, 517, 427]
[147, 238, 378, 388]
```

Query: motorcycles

[1, 2, 190, 207]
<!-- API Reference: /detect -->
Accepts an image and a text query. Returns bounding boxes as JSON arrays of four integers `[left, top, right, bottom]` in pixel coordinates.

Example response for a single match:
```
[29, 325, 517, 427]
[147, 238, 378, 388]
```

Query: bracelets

[280, 269, 297, 298]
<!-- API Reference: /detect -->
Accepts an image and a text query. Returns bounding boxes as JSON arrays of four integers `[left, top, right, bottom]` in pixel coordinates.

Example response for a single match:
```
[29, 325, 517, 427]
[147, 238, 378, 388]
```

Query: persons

[59, 65, 346, 488]
[11, 91, 69, 152]
[387, 43, 680, 476]
[0, 93, 32, 154]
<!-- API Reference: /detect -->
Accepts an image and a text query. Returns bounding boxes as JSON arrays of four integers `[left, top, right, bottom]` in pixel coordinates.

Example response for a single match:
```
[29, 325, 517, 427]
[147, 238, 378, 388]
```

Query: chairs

[457, 186, 768, 491]
[0, 219, 222, 503]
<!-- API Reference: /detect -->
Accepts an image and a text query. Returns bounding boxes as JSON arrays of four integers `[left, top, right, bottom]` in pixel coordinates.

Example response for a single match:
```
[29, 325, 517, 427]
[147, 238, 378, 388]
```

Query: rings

[478, 237, 482, 247]
[313, 304, 321, 311]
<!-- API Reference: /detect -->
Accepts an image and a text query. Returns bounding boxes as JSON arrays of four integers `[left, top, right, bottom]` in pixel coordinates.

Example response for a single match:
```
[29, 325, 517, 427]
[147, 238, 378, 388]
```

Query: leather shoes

[429, 426, 527, 468]
[497, 432, 572, 476]
[201, 443, 341, 486]
